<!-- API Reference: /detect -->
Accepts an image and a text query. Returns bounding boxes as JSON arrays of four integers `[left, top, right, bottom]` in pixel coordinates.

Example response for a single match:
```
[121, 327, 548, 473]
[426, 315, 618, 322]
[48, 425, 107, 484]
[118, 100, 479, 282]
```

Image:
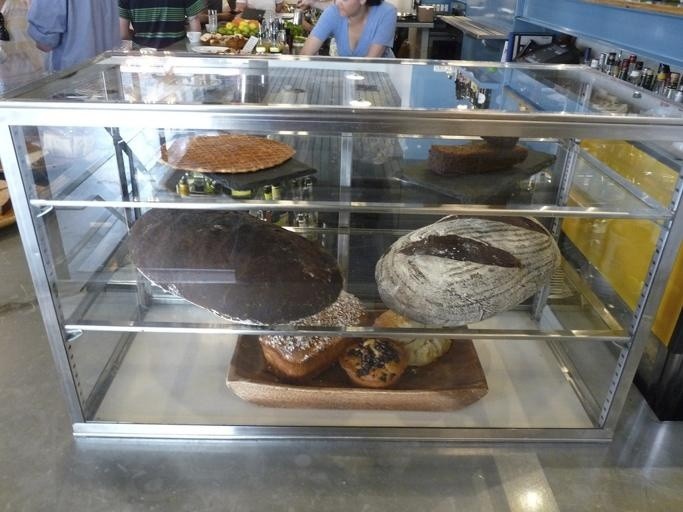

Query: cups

[205, 24, 217, 32]
[186, 31, 201, 43]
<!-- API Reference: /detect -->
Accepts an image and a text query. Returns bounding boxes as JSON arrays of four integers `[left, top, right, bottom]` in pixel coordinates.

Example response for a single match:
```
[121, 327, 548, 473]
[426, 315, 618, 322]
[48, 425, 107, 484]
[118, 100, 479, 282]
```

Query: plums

[218, 18, 260, 38]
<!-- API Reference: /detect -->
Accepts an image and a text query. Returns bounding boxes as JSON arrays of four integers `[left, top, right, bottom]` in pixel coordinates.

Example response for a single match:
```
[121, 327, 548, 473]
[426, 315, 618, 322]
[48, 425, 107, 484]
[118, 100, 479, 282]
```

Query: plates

[191, 46, 229, 53]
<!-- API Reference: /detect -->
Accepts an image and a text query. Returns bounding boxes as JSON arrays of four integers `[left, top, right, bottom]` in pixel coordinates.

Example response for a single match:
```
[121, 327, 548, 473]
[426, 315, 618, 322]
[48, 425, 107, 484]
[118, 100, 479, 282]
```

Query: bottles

[455, 71, 491, 109]
[583, 47, 683, 115]
[175, 171, 321, 244]
[208, 10, 217, 34]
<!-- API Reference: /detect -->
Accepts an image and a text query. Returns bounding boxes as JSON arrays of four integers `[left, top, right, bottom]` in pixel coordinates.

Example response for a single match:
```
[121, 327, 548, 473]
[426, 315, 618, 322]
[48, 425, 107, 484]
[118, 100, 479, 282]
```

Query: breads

[258, 290, 367, 386]
[373, 308, 452, 366]
[429, 136, 530, 176]
[338, 338, 409, 387]
[128, 209, 343, 326]
[375, 216, 561, 327]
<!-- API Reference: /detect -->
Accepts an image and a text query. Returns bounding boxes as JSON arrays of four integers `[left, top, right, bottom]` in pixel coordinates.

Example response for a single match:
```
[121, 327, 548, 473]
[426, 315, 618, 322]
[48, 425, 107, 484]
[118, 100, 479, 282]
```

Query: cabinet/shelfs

[1, 49, 682, 447]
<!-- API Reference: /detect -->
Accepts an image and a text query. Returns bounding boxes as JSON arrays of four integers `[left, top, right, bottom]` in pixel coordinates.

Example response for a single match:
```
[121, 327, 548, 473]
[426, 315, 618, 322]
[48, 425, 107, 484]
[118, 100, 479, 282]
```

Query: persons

[27, 1, 120, 74]
[298, 1, 397, 59]
[118, 1, 201, 52]
[245, 0, 283, 13]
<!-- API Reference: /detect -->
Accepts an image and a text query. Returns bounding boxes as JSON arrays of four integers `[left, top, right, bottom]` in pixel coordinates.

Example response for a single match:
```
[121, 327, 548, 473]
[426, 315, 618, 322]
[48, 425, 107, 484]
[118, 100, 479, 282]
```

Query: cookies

[152, 132, 296, 173]
